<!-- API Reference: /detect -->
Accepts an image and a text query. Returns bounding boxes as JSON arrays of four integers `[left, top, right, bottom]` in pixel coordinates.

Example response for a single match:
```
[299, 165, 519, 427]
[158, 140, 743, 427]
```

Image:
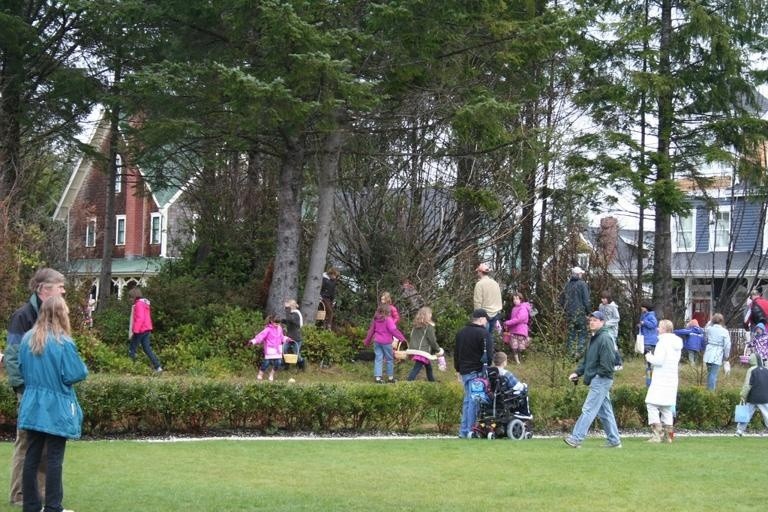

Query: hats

[475, 264, 489, 273]
[572, 267, 585, 273]
[473, 309, 489, 320]
[588, 311, 604, 320]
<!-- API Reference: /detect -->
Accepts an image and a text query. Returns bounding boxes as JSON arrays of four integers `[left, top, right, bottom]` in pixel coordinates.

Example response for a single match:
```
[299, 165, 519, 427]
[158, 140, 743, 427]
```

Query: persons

[733, 352, 768, 438]
[559, 266, 594, 357]
[745, 323, 768, 367]
[126, 285, 165, 376]
[701, 312, 731, 392]
[598, 290, 625, 373]
[405, 305, 446, 382]
[247, 312, 293, 381]
[473, 263, 503, 344]
[562, 309, 623, 449]
[645, 319, 683, 442]
[364, 302, 406, 383]
[493, 349, 531, 416]
[276, 297, 308, 372]
[741, 289, 768, 334]
[381, 290, 402, 365]
[503, 291, 532, 366]
[454, 309, 495, 439]
[673, 318, 706, 367]
[635, 299, 658, 368]
[319, 266, 341, 333]
[6, 268, 67, 506]
[403, 280, 426, 313]
[19, 293, 87, 512]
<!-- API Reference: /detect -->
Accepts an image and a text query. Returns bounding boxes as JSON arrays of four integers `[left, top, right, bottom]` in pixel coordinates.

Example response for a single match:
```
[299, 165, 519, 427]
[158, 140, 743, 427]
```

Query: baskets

[394, 340, 409, 359]
[739, 344, 750, 364]
[283, 339, 299, 364]
[316, 301, 327, 320]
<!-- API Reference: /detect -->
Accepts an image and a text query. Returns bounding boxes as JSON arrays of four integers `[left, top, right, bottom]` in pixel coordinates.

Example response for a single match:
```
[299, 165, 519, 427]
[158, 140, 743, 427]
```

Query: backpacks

[464, 366, 493, 405]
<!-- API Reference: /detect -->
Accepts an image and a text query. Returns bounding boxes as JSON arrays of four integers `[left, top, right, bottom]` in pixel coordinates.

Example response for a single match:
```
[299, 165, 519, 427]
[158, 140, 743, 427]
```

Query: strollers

[474, 365, 534, 445]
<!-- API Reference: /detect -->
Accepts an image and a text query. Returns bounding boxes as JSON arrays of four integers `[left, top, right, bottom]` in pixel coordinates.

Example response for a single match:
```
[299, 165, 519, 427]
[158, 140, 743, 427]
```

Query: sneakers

[601, 443, 622, 448]
[376, 379, 386, 383]
[563, 438, 581, 448]
[388, 378, 398, 382]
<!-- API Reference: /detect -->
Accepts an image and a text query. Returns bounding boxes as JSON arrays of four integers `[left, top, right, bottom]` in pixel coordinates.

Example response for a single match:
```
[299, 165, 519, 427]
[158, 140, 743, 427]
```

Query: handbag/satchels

[634, 327, 644, 354]
[504, 324, 510, 344]
[723, 361, 731, 377]
[735, 400, 750, 423]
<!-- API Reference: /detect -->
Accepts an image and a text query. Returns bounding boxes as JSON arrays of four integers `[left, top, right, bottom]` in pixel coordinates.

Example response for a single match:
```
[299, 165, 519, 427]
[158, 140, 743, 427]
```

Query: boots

[297, 360, 306, 370]
[257, 370, 263, 381]
[643, 424, 663, 442]
[663, 425, 673, 444]
[269, 369, 275, 381]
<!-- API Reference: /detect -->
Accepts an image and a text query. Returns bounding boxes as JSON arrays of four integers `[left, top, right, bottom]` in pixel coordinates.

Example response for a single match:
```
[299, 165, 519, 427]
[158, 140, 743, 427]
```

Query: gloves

[568, 373, 577, 381]
